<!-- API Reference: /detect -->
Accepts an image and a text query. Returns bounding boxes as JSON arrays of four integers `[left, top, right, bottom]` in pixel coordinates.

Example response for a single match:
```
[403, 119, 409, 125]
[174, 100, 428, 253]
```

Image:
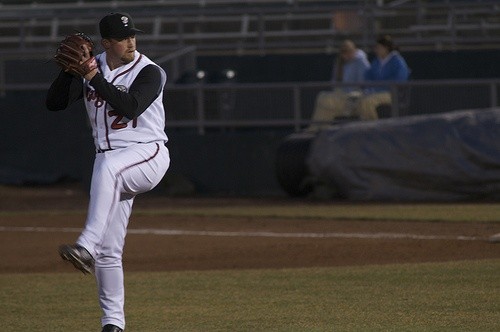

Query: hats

[99, 13, 143, 40]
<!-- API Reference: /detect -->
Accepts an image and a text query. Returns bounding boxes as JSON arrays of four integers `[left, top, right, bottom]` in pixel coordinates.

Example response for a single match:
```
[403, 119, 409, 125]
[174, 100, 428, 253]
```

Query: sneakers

[101, 323, 124, 332]
[59, 243, 95, 275]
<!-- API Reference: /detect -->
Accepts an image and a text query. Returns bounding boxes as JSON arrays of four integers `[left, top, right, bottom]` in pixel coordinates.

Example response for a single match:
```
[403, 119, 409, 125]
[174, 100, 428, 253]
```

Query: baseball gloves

[49, 31, 104, 91]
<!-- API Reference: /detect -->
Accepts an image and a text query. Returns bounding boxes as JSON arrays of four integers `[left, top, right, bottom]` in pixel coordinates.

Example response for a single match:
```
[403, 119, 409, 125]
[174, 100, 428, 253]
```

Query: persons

[303, 39, 371, 131]
[358, 35, 409, 122]
[45, 13, 171, 332]
